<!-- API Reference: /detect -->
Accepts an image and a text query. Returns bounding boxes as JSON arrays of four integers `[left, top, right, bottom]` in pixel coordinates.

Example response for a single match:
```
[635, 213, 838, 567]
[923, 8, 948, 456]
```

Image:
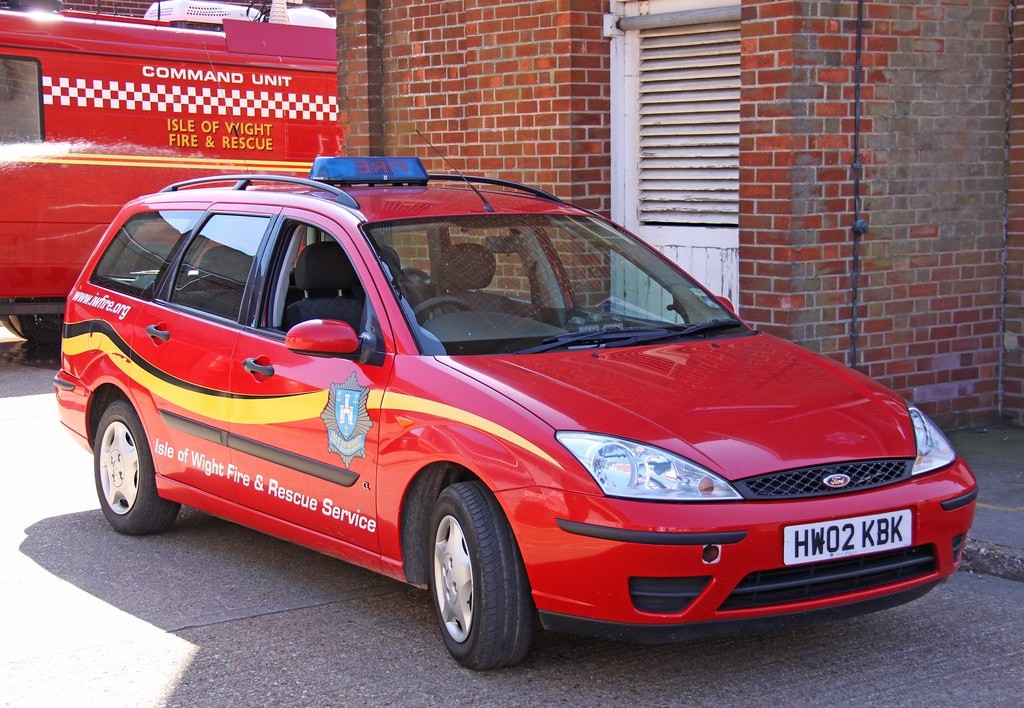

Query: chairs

[287, 241, 363, 345]
[432, 243, 521, 317]
[196, 244, 255, 320]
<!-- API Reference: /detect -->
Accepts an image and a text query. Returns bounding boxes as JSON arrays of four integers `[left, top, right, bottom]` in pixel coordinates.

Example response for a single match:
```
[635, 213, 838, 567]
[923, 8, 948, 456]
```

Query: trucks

[1, 0, 341, 343]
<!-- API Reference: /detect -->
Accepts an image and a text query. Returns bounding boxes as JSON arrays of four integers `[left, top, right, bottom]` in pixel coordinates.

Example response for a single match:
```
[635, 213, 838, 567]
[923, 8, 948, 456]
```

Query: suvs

[52, 157, 977, 672]
